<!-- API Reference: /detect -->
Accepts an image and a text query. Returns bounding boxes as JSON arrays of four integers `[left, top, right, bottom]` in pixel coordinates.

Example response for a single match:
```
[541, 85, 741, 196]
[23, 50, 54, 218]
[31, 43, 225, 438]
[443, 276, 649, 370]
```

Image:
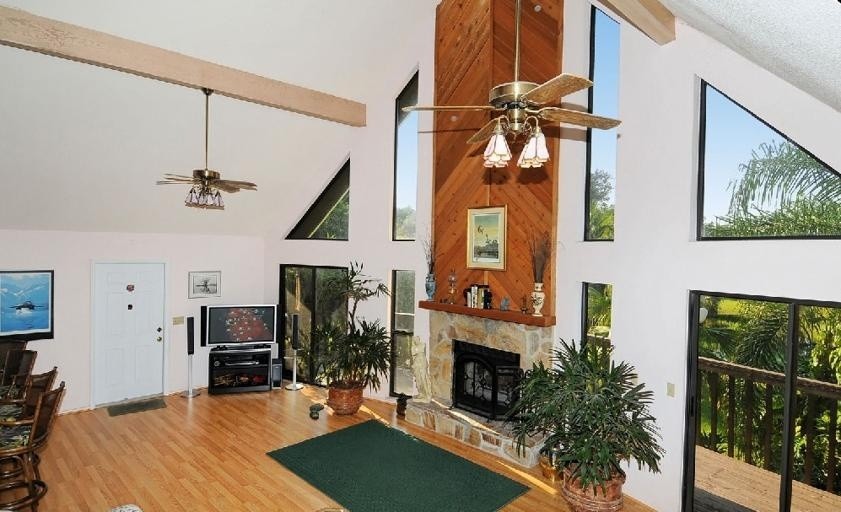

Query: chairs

[1, 381, 65, 512]
[1, 341, 26, 352]
[0, 366, 60, 478]
[1, 351, 37, 400]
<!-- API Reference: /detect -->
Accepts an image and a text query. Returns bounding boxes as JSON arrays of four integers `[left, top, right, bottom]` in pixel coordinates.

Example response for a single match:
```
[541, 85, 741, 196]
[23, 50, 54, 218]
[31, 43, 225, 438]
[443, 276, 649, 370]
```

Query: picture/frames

[1, 270, 54, 338]
[466, 204, 509, 270]
[188, 271, 220, 298]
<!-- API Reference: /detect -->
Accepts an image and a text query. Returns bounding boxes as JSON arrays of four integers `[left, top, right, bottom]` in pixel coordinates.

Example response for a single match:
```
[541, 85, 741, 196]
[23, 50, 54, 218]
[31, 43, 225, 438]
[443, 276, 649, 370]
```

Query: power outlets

[173, 317, 184, 325]
[666, 382, 675, 397]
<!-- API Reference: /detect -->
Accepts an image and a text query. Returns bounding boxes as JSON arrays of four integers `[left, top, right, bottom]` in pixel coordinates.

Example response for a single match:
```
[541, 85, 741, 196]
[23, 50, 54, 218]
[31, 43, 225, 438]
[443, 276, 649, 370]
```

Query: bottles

[467, 284, 489, 309]
[448, 267, 457, 305]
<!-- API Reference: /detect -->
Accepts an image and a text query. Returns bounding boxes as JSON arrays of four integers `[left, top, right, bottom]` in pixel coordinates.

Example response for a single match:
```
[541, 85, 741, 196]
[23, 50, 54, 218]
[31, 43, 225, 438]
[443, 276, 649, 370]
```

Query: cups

[500, 296, 510, 311]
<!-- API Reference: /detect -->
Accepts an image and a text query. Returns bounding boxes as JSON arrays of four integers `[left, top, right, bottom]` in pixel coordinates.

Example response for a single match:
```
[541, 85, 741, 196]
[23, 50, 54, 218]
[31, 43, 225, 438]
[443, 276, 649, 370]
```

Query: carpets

[268, 418, 529, 512]
[105, 504, 141, 512]
[108, 399, 167, 416]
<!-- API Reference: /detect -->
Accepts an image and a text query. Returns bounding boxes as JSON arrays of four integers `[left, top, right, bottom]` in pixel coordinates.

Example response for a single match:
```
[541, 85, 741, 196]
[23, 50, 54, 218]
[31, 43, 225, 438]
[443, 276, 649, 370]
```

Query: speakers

[285, 315, 304, 390]
[181, 317, 201, 398]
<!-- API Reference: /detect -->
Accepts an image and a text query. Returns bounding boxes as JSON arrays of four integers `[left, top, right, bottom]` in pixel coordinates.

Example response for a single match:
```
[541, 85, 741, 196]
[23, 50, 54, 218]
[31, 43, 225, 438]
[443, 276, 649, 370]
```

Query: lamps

[481, 115, 550, 168]
[400, 1, 622, 169]
[155, 88, 258, 210]
[184, 187, 226, 211]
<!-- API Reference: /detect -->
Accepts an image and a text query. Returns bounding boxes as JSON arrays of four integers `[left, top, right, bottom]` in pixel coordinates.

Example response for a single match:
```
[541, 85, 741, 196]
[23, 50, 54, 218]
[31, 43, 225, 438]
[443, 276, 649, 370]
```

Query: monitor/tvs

[206, 304, 278, 347]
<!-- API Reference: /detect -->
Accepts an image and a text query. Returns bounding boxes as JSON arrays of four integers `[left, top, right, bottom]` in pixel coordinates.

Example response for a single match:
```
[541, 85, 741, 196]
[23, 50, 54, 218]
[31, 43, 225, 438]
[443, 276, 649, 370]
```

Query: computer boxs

[271, 359, 283, 389]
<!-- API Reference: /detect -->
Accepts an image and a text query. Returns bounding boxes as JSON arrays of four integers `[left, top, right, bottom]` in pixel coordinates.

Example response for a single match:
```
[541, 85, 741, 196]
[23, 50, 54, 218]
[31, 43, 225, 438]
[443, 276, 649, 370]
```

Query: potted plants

[501, 331, 659, 509]
[519, 363, 564, 480]
[314, 269, 399, 415]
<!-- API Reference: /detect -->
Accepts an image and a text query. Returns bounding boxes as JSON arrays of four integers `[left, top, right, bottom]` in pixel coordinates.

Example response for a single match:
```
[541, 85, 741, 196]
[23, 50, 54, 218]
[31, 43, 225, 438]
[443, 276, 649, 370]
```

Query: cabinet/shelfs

[208, 347, 282, 392]
